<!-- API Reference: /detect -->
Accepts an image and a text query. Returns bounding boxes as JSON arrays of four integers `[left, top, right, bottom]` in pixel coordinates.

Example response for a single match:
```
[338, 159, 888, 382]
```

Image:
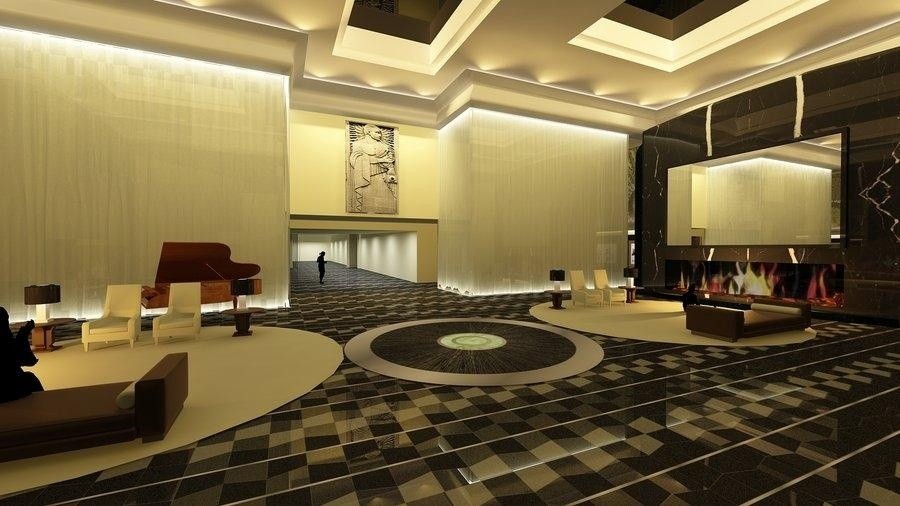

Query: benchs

[0, 351, 189, 465]
[685, 299, 813, 344]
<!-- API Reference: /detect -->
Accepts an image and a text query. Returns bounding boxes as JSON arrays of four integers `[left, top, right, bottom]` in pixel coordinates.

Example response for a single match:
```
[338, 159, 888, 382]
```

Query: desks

[545, 290, 571, 309]
[222, 307, 266, 337]
[12, 317, 76, 353]
[618, 286, 646, 304]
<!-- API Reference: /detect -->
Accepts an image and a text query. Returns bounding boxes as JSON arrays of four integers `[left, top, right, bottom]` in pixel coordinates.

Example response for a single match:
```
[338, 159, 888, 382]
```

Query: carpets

[0, 324, 346, 498]
[528, 300, 819, 346]
[344, 317, 607, 388]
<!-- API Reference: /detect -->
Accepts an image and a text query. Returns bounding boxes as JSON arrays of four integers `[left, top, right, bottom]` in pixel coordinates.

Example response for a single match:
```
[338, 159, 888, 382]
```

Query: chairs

[569, 269, 628, 308]
[80, 281, 203, 353]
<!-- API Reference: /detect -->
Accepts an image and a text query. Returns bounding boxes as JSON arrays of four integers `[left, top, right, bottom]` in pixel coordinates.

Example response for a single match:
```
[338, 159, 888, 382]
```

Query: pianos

[140, 241, 262, 310]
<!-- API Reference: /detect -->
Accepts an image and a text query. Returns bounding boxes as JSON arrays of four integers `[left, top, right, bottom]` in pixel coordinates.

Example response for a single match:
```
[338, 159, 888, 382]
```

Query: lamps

[623, 267, 639, 287]
[549, 270, 566, 291]
[25, 283, 62, 324]
[230, 278, 263, 310]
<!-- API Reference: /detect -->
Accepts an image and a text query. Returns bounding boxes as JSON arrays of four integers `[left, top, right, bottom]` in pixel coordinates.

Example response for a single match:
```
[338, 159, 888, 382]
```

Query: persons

[348, 123, 397, 214]
[0, 306, 44, 403]
[317, 251, 328, 285]
